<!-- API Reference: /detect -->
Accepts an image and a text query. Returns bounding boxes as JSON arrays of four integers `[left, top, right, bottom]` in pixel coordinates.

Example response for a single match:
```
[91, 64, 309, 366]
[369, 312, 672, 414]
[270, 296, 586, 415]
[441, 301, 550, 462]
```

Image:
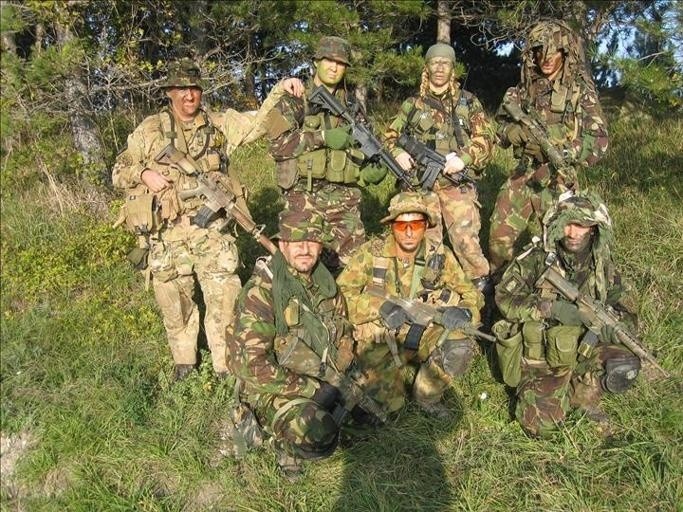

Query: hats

[268, 209, 334, 244]
[551, 197, 598, 227]
[310, 35, 352, 68]
[156, 60, 205, 90]
[379, 191, 438, 228]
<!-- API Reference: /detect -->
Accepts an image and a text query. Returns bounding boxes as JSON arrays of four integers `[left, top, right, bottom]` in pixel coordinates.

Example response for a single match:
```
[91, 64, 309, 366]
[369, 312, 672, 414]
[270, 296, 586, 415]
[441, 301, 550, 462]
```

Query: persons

[225, 210, 373, 484]
[337, 193, 485, 422]
[494, 191, 641, 440]
[109, 61, 306, 384]
[487, 17, 609, 278]
[266, 36, 388, 270]
[383, 44, 490, 291]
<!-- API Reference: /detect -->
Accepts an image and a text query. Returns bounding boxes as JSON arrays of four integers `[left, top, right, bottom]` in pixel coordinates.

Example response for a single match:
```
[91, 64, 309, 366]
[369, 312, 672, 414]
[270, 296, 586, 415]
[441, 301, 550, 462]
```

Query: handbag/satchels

[514, 356, 574, 438]
[124, 193, 165, 235]
[491, 318, 523, 388]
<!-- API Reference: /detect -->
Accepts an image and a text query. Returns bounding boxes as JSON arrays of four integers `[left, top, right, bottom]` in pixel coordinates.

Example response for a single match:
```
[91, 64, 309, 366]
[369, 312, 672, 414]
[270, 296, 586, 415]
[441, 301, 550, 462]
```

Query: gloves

[362, 163, 388, 186]
[596, 324, 622, 345]
[505, 122, 528, 146]
[394, 151, 417, 173]
[324, 125, 360, 150]
[311, 380, 342, 413]
[441, 306, 468, 331]
[523, 142, 548, 163]
[550, 300, 582, 326]
[442, 152, 466, 175]
[382, 305, 415, 331]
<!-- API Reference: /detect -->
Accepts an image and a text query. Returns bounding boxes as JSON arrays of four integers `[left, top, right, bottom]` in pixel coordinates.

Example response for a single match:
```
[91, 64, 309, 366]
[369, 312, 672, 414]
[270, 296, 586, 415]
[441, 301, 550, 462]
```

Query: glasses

[390, 219, 426, 232]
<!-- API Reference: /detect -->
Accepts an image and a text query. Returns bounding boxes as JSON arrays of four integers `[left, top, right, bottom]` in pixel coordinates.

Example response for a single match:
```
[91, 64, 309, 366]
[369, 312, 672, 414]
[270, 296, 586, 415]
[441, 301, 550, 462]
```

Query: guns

[308, 83, 414, 191]
[154, 144, 278, 256]
[534, 268, 671, 380]
[500, 101, 574, 186]
[367, 286, 497, 350]
[279, 339, 389, 426]
[396, 129, 475, 188]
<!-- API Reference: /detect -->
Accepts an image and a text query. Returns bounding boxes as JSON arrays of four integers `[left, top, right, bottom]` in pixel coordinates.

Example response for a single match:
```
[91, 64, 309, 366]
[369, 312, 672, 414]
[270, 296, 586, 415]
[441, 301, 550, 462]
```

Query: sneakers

[217, 370, 230, 382]
[277, 449, 309, 484]
[569, 390, 608, 423]
[174, 364, 194, 381]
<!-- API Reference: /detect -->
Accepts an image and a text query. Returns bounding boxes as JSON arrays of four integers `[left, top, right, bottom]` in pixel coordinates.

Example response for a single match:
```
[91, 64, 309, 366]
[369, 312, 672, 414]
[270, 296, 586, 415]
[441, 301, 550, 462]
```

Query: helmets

[526, 21, 574, 54]
